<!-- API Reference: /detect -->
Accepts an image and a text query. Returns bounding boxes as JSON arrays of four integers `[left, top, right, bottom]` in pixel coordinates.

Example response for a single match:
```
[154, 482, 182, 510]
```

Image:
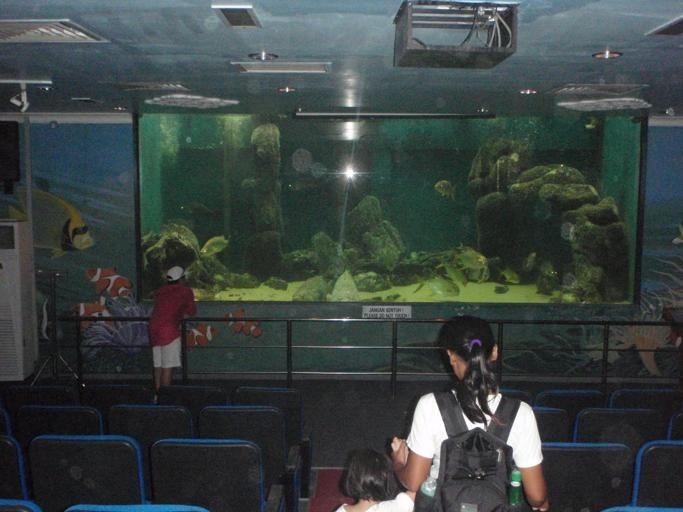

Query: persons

[391, 316, 549, 511]
[333, 436, 416, 511]
[146, 266, 196, 404]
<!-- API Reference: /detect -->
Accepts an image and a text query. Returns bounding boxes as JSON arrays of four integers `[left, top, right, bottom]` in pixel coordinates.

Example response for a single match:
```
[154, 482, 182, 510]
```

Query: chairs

[498, 384, 682, 512]
[1, 380, 322, 512]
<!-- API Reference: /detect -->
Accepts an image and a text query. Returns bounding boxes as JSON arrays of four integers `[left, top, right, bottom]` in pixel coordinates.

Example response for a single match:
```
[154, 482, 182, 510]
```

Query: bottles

[420, 476, 435, 496]
[508, 468, 521, 505]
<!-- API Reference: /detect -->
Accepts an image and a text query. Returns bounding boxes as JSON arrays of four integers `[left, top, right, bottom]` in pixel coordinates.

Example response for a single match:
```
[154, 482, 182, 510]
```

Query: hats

[166, 267, 183, 280]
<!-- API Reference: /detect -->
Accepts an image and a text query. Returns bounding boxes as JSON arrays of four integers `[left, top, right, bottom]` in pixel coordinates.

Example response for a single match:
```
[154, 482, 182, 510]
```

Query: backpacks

[413, 390, 530, 512]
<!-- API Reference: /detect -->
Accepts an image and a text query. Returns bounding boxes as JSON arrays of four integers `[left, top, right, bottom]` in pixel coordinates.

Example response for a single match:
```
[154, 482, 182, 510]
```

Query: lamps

[0, 217, 38, 381]
